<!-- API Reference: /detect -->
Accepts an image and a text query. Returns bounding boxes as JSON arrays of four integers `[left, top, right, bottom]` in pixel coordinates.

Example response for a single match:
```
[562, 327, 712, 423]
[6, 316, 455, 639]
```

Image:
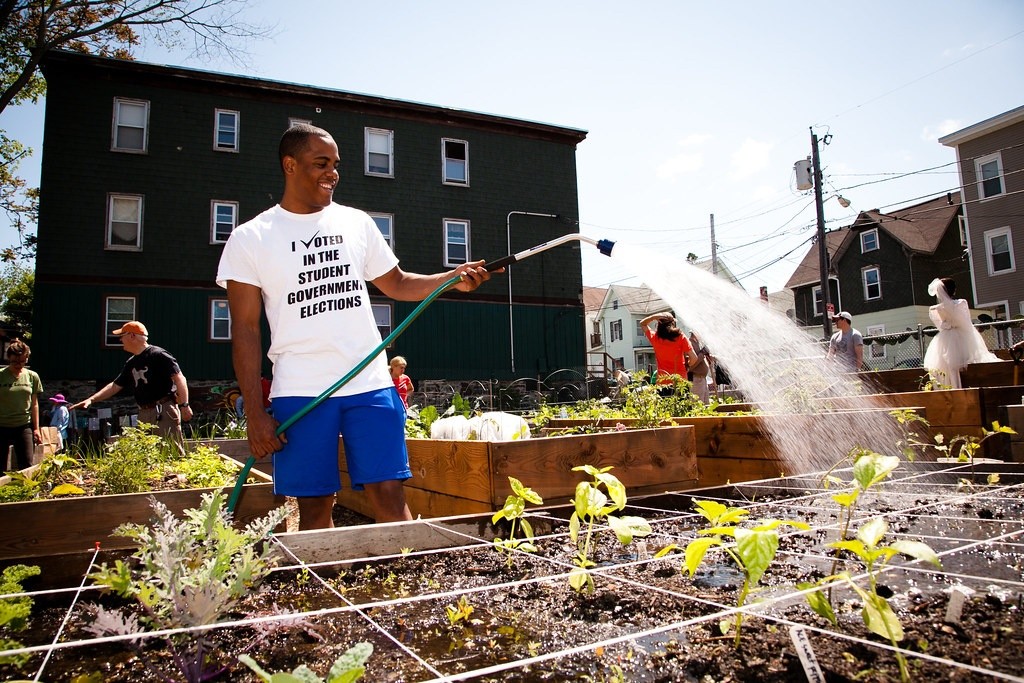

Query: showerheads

[462, 232, 617, 280]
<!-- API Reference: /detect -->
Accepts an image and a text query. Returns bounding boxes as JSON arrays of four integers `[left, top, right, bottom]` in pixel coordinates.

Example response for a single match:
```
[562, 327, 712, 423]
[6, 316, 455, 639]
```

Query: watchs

[178, 403, 189, 407]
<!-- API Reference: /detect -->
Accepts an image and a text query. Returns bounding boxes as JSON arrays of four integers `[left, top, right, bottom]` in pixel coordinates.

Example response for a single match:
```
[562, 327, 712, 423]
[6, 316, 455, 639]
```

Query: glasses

[9, 358, 28, 365]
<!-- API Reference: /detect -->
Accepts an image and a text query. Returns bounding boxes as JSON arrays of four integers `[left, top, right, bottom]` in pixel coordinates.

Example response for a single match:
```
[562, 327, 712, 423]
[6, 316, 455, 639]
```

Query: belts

[142, 396, 170, 409]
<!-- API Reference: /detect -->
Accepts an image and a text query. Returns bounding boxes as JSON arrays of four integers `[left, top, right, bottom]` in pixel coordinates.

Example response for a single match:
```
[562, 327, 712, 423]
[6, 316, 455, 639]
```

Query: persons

[640, 313, 698, 410]
[388, 356, 414, 409]
[70, 321, 193, 456]
[924, 278, 1003, 390]
[0, 338, 43, 477]
[215, 125, 505, 531]
[687, 329, 710, 406]
[49, 394, 78, 454]
[827, 311, 864, 373]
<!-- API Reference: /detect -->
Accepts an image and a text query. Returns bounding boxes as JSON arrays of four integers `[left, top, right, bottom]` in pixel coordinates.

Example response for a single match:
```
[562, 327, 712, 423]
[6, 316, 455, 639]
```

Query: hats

[49, 394, 68, 404]
[832, 311, 851, 321]
[112, 321, 149, 336]
